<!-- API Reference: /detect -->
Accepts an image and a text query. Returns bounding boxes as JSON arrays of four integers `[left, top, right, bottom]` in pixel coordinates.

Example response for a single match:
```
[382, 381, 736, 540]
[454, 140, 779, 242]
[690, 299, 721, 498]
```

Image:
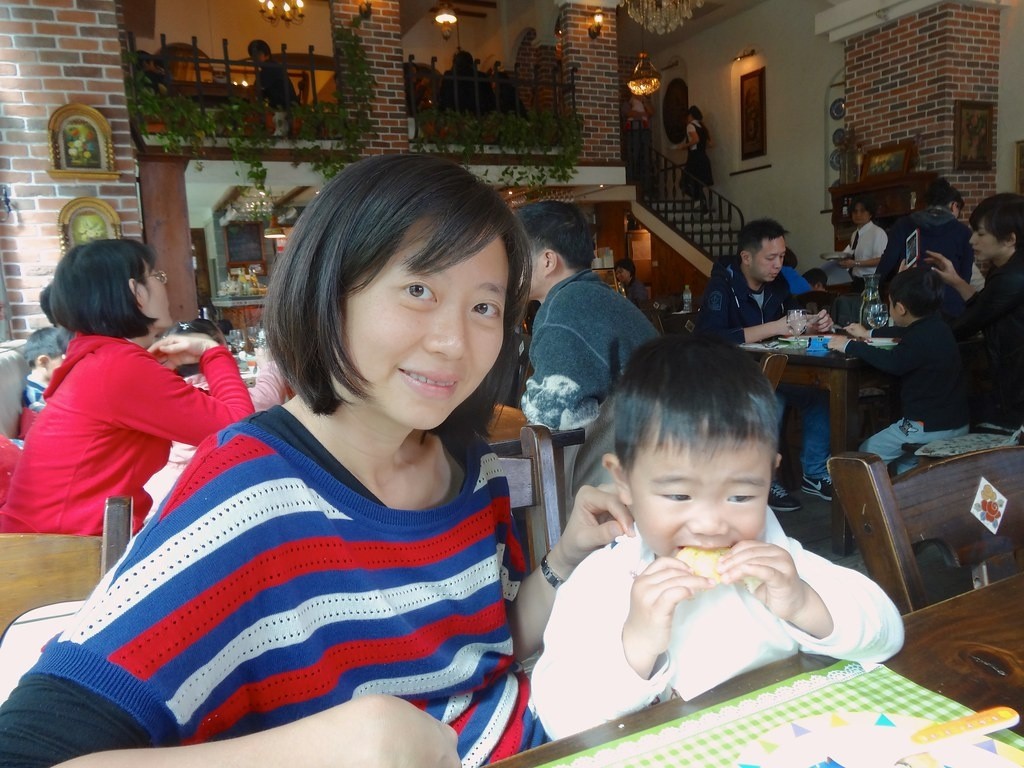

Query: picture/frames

[953, 101, 995, 175]
[57, 198, 124, 256]
[859, 140, 913, 179]
[905, 228, 920, 271]
[740, 65, 768, 162]
[47, 104, 120, 184]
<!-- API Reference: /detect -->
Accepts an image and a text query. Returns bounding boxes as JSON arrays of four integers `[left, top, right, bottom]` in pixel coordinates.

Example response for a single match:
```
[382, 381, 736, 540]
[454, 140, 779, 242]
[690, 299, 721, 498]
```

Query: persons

[514, 200, 665, 432]
[677, 104, 715, 217]
[403, 62, 427, 117]
[0, 150, 637, 768]
[826, 264, 970, 476]
[439, 50, 496, 122]
[0, 238, 285, 534]
[135, 48, 168, 97]
[247, 39, 300, 111]
[530, 331, 908, 742]
[698, 180, 982, 514]
[924, 193, 1024, 431]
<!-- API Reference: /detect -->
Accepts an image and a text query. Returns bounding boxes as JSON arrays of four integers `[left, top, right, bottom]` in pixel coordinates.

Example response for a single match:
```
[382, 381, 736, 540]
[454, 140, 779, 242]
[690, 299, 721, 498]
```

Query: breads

[677, 546, 761, 593]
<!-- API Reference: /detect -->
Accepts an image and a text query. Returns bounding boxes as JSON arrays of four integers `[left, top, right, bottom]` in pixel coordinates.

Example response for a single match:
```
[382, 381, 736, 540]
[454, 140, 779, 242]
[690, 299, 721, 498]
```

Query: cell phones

[805, 314, 822, 326]
[906, 228, 920, 269]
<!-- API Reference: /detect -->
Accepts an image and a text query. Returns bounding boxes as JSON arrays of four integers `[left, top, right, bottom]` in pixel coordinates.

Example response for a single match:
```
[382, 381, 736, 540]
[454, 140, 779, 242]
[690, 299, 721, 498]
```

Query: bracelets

[539, 549, 566, 590]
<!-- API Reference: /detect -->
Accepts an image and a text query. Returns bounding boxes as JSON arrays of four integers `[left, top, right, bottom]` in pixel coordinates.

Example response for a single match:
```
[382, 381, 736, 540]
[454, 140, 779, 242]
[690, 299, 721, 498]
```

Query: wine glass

[229, 330, 244, 353]
[786, 310, 807, 348]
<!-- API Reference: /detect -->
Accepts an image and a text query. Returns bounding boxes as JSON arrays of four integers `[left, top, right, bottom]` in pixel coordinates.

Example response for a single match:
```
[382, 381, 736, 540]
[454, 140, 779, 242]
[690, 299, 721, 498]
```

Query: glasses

[134, 269, 168, 286]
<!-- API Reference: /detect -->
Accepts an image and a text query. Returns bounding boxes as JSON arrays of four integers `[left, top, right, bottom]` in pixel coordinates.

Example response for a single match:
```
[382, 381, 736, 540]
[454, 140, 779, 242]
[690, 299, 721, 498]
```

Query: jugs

[860, 274, 882, 328]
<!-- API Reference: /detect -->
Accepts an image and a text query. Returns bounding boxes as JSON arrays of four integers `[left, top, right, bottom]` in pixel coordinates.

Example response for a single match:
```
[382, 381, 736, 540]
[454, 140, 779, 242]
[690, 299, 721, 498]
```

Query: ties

[847, 231, 861, 274]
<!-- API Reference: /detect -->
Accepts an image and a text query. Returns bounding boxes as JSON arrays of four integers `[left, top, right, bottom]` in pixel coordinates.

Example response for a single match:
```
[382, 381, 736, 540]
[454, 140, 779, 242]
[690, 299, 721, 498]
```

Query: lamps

[257, 0, 305, 27]
[435, 1, 457, 41]
[620, 1, 704, 34]
[587, 7, 604, 40]
[626, 23, 660, 96]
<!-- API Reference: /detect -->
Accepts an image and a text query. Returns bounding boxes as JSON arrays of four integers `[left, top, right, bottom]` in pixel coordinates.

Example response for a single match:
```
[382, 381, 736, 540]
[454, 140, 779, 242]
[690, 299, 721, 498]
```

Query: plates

[734, 711, 1023, 768]
[670, 144, 677, 149]
[864, 338, 898, 350]
[820, 253, 851, 260]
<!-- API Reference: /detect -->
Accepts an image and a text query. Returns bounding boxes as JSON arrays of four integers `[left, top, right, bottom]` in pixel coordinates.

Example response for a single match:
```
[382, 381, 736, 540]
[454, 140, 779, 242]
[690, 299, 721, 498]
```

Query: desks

[174, 80, 258, 137]
[739, 335, 904, 555]
[489, 570, 1024, 767]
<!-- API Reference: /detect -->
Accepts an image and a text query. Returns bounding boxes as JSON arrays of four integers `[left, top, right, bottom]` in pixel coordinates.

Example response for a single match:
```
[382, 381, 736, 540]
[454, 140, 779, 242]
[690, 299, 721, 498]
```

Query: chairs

[826, 439, 1024, 614]
[518, 422, 588, 573]
[0, 493, 131, 637]
[265, 71, 310, 134]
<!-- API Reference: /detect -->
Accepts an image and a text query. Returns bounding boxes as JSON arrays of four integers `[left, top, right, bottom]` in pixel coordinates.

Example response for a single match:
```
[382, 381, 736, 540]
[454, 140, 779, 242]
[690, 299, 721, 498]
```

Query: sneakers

[768, 482, 802, 511]
[800, 476, 832, 502]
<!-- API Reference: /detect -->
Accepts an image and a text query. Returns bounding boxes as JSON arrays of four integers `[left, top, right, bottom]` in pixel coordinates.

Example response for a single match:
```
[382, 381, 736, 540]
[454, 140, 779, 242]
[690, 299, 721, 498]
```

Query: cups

[866, 304, 888, 328]
[248, 327, 266, 348]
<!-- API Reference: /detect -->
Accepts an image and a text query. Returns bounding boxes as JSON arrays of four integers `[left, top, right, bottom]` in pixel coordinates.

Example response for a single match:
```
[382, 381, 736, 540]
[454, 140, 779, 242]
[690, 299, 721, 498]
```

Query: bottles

[683, 284, 691, 312]
[778, 334, 848, 348]
[618, 282, 626, 299]
